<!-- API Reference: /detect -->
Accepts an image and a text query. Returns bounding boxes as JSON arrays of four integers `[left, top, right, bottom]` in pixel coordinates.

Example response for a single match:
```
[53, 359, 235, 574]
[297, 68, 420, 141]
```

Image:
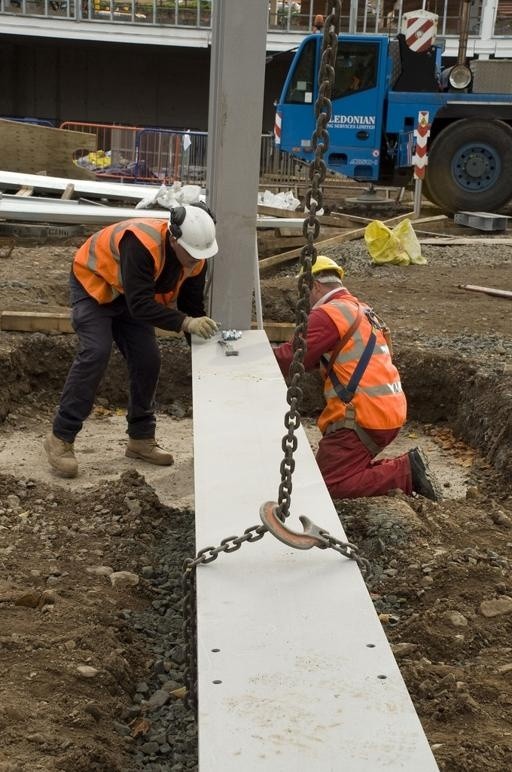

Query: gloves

[182, 315, 222, 339]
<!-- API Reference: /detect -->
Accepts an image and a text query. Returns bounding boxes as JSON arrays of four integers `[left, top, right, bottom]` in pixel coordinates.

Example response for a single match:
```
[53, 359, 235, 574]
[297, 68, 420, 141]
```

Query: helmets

[168, 206, 218, 261]
[297, 255, 344, 282]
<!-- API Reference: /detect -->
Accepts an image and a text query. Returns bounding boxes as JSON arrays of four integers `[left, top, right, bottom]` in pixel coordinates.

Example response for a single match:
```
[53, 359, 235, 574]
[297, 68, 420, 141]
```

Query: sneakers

[124, 435, 174, 466]
[409, 446, 443, 502]
[44, 432, 78, 479]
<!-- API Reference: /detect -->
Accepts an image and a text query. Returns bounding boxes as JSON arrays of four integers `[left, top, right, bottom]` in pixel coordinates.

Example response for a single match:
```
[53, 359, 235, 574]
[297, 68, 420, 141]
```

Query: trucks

[273, 33, 512, 218]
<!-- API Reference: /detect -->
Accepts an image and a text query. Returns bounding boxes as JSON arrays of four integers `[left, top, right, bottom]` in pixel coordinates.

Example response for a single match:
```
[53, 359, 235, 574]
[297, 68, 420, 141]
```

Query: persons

[270, 254, 445, 503]
[40, 200, 224, 479]
